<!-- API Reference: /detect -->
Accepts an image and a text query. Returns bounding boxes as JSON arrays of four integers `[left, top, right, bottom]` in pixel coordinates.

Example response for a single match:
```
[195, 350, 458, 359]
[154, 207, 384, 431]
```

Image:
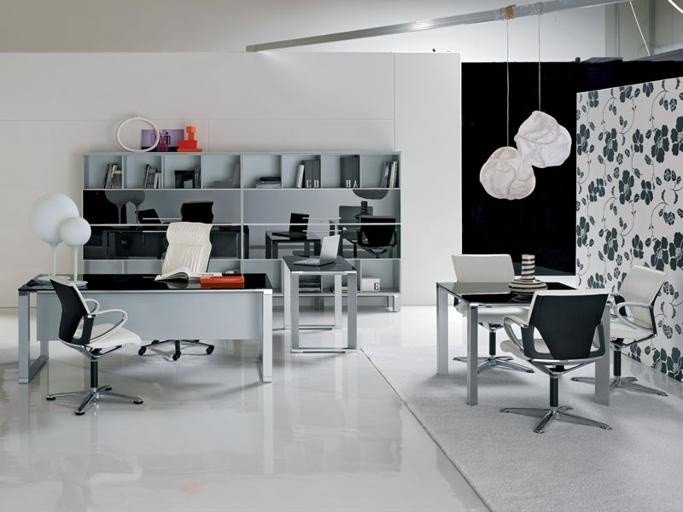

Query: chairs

[138, 221, 215, 362]
[452, 254, 667, 435]
[45, 279, 144, 416]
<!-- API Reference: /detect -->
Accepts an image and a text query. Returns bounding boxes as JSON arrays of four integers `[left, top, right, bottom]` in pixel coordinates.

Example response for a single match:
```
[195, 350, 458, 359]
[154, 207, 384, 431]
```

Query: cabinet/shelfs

[82, 152, 403, 312]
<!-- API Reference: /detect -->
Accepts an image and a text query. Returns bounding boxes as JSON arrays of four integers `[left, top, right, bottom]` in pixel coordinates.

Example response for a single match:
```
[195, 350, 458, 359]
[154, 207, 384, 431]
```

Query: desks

[18, 273, 274, 384]
[436, 282, 613, 406]
[281, 254, 358, 352]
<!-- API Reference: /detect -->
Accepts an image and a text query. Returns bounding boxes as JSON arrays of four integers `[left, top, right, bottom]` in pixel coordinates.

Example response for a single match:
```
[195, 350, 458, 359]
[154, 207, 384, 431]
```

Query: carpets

[361, 343, 682, 512]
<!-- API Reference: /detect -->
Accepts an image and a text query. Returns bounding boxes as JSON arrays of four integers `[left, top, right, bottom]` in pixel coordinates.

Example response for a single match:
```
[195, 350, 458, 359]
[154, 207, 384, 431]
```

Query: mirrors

[83, 190, 401, 258]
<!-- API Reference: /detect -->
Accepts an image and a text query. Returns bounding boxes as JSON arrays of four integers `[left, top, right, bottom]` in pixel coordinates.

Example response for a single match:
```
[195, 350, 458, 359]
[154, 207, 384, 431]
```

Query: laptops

[293, 235, 340, 266]
[272, 213, 309, 237]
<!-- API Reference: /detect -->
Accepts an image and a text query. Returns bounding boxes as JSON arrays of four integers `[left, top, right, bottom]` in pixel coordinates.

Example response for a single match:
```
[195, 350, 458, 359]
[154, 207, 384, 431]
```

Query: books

[153, 266, 223, 280]
[377, 160, 389, 188]
[254, 176, 281, 189]
[388, 160, 398, 189]
[340, 156, 359, 189]
[102, 161, 160, 189]
[295, 156, 320, 189]
[194, 166, 200, 188]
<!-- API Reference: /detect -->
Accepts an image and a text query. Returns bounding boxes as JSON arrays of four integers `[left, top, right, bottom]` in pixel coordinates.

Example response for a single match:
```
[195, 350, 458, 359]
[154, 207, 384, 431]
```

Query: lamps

[479, 14, 573, 200]
[29, 193, 91, 286]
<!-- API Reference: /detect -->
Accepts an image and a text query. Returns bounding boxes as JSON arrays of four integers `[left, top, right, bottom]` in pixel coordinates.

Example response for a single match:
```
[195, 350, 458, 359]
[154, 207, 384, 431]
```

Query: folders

[340, 156, 360, 187]
[302, 160, 320, 188]
